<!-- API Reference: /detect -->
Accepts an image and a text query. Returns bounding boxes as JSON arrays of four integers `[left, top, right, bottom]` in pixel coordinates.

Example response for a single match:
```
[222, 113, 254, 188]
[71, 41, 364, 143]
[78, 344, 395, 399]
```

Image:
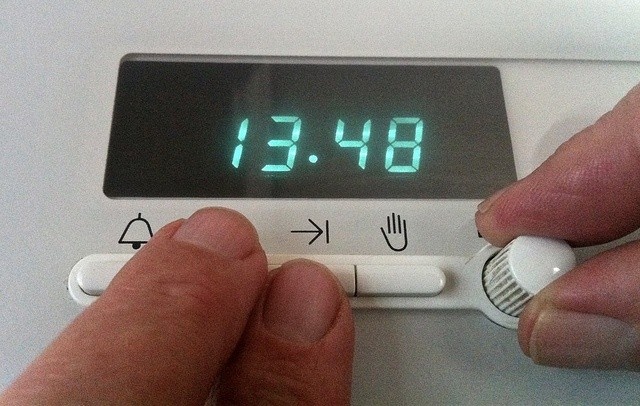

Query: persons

[3, 84, 639, 404]
